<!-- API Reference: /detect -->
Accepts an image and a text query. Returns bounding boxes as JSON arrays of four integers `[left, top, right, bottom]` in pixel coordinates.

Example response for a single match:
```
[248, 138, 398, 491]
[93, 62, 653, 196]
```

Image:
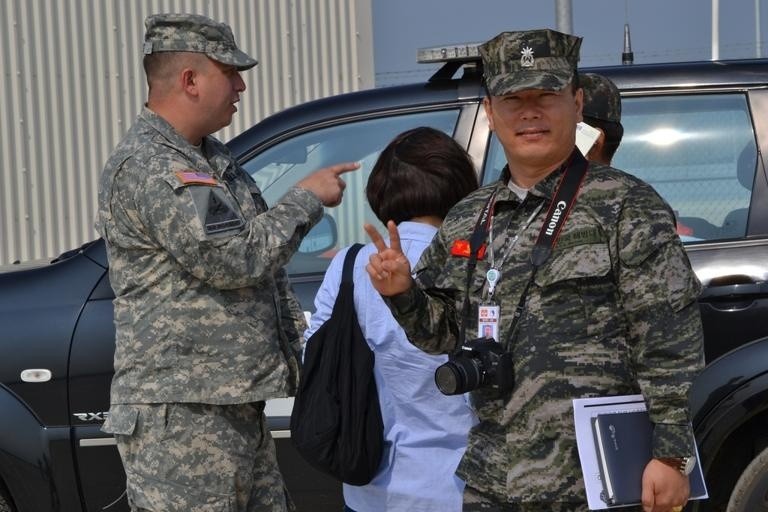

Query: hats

[577, 72, 622, 124]
[476, 29, 584, 97]
[143, 12, 258, 71]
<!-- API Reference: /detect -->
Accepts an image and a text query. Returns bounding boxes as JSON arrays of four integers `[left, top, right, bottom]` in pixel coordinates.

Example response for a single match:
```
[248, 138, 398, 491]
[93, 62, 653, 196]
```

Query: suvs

[1, 23, 768, 512]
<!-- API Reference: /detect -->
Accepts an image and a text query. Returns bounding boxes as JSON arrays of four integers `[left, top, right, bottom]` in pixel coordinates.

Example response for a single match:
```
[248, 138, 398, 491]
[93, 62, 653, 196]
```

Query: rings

[671, 504, 683, 512]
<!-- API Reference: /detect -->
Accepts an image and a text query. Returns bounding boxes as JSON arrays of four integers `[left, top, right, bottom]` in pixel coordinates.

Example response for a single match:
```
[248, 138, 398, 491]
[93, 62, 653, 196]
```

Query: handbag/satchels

[291, 282, 384, 486]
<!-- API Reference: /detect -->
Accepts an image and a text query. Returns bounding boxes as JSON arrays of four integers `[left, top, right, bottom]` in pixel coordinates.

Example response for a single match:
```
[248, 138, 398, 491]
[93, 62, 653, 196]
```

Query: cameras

[435, 337, 514, 396]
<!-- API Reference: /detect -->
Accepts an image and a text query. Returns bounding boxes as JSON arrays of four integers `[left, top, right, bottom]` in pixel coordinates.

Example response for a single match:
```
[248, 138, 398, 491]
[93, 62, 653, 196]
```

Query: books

[591, 410, 706, 507]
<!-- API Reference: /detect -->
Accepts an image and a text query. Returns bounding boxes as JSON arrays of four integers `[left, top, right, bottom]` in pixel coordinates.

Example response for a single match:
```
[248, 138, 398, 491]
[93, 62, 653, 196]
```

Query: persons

[301, 124, 487, 511]
[94, 12, 362, 512]
[363, 28, 705, 512]
[485, 326, 491, 338]
[577, 70, 625, 170]
